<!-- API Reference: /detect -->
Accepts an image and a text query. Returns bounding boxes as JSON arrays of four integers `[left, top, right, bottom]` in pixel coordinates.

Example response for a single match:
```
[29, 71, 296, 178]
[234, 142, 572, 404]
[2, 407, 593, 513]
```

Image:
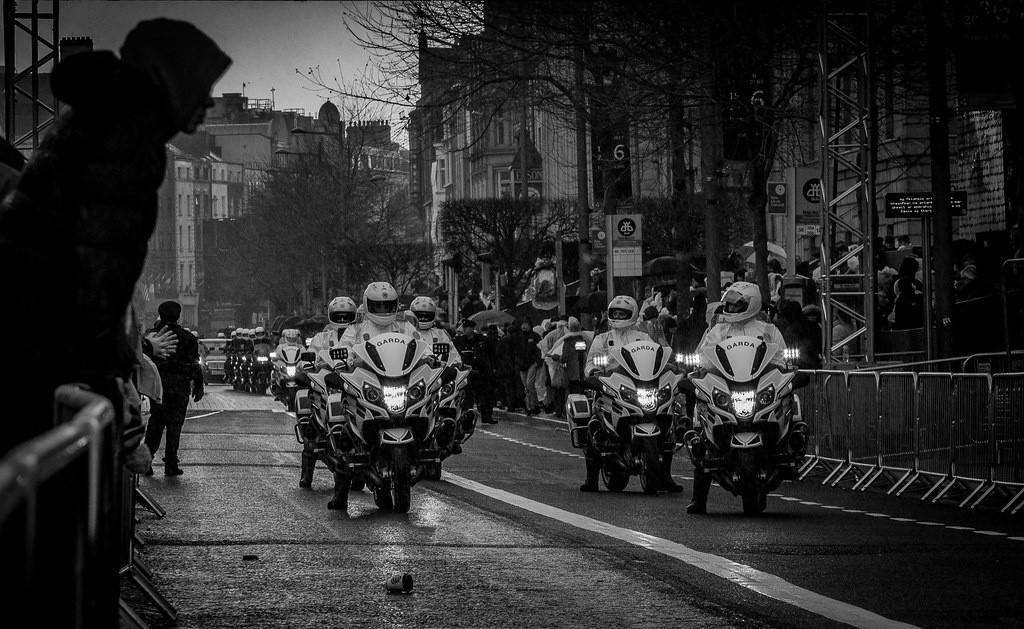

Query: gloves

[192, 387, 204, 402]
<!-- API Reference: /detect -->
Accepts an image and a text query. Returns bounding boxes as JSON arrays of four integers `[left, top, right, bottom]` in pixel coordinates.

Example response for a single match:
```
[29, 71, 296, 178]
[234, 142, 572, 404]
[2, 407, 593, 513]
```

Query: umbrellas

[140, 349, 165, 407]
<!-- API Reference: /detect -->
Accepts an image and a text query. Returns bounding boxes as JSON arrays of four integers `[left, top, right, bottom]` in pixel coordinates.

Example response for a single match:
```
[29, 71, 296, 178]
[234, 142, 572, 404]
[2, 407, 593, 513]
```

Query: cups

[385, 574, 413, 592]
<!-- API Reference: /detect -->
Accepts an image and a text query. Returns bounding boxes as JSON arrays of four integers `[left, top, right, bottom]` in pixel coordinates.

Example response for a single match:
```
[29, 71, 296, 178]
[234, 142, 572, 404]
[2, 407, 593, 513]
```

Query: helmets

[191, 331, 198, 337]
[230, 327, 265, 339]
[720, 282, 762, 323]
[607, 295, 638, 329]
[410, 296, 438, 329]
[184, 328, 190, 332]
[364, 282, 399, 325]
[285, 329, 298, 346]
[328, 296, 357, 331]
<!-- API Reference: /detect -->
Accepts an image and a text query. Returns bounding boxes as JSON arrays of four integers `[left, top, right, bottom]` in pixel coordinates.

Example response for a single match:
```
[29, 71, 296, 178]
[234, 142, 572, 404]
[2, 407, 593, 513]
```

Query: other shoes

[165, 467, 184, 476]
[546, 409, 554, 413]
[481, 417, 498, 424]
[580, 484, 599, 491]
[300, 477, 312, 488]
[532, 409, 540, 414]
[328, 498, 348, 509]
[557, 413, 562, 418]
[687, 501, 707, 513]
[659, 479, 684, 492]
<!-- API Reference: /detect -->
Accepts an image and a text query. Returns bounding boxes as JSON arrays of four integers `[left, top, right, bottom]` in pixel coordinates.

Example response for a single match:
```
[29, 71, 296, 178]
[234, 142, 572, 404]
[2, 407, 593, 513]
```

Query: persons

[871, 257, 977, 326]
[722, 234, 914, 359]
[321, 280, 436, 511]
[687, 281, 796, 513]
[632, 276, 707, 353]
[184, 324, 273, 384]
[145, 300, 204, 476]
[834, 318, 852, 355]
[439, 314, 579, 425]
[123, 299, 179, 396]
[298, 291, 357, 490]
[410, 294, 464, 455]
[588, 310, 607, 333]
[579, 295, 681, 492]
[274, 327, 307, 402]
[0, 17, 231, 629]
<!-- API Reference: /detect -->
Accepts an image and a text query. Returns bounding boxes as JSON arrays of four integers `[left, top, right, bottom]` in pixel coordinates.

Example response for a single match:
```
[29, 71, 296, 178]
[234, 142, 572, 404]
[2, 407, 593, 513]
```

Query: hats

[461, 319, 476, 327]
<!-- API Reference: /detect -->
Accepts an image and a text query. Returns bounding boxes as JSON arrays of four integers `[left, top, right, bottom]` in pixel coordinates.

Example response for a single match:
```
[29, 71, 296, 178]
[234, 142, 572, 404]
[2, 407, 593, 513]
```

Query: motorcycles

[563, 339, 693, 498]
[217, 331, 481, 515]
[675, 336, 811, 519]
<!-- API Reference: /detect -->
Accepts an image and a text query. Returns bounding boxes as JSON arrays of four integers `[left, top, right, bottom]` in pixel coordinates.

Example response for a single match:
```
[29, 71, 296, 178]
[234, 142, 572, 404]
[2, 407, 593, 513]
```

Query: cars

[198, 337, 233, 378]
